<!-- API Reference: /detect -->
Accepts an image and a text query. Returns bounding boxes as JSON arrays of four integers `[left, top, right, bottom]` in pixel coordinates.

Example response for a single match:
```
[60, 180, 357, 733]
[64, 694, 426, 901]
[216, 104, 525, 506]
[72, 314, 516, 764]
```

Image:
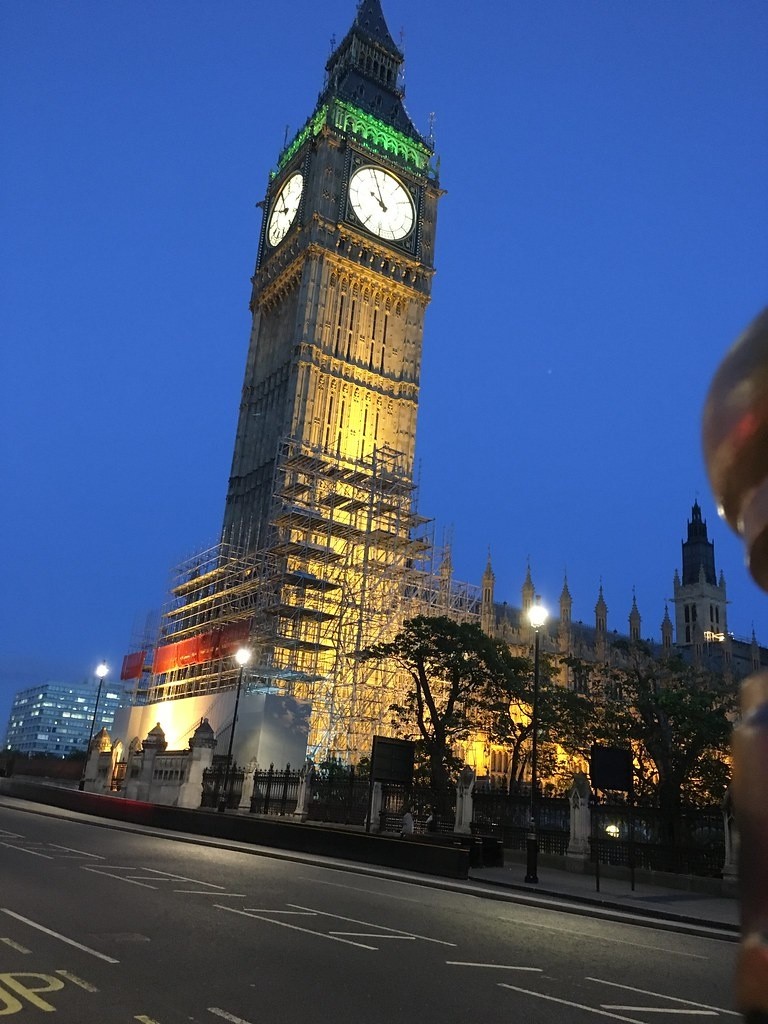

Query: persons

[425, 809, 436, 833]
[216, 784, 227, 812]
[400, 807, 414, 834]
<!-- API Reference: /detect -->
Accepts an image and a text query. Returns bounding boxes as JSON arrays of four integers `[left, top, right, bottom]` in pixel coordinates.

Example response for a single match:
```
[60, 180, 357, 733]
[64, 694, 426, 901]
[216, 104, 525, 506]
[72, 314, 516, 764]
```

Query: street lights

[217, 648, 252, 810]
[78, 662, 109, 790]
[525, 604, 548, 885]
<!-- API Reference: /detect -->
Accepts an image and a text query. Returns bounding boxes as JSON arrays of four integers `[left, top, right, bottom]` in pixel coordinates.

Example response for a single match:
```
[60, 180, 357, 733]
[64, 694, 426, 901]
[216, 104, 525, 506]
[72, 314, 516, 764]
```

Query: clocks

[350, 169, 414, 240]
[269, 174, 303, 247]
